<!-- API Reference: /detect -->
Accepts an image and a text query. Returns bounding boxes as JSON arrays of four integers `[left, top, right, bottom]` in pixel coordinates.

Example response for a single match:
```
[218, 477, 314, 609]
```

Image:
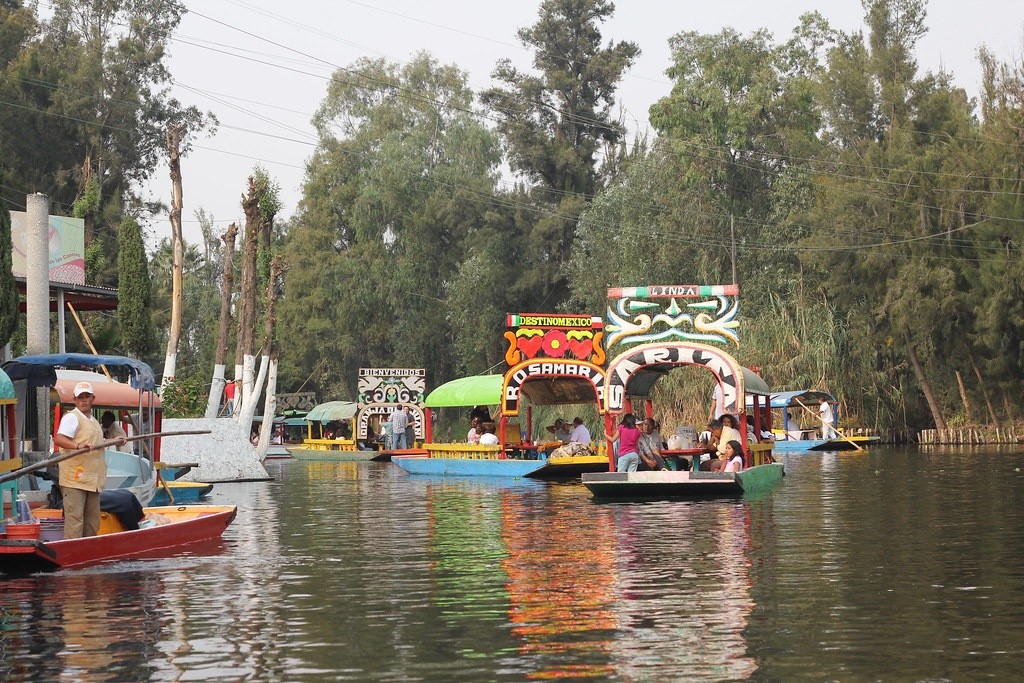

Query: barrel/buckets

[32, 509, 63, 520]
[39, 518, 64, 541]
[6, 525, 40, 540]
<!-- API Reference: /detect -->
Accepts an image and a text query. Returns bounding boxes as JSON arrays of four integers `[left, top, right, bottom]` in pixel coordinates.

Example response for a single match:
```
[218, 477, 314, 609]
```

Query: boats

[743, 389, 881, 453]
[0, 350, 238, 569]
[285, 369, 428, 462]
[248, 391, 319, 459]
[390, 312, 609, 479]
[579, 284, 788, 498]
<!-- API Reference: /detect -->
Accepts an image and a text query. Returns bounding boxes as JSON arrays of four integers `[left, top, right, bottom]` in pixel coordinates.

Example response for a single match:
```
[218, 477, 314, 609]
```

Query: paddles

[0, 429, 214, 483]
[795, 398, 864, 451]
[67, 300, 175, 501]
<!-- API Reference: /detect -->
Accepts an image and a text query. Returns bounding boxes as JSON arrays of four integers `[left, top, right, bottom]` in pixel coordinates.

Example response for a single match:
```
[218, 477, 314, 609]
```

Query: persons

[545, 417, 591, 447]
[466, 405, 503, 458]
[251, 428, 304, 444]
[699, 414, 775, 472]
[604, 414, 688, 473]
[708, 382, 735, 422]
[222, 380, 235, 415]
[787, 413, 799, 430]
[813, 398, 836, 439]
[323, 425, 351, 440]
[368, 404, 415, 450]
[102, 412, 132, 454]
[54, 382, 127, 538]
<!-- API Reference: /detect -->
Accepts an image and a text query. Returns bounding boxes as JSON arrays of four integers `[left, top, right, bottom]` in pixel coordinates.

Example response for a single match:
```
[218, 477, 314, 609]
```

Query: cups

[534, 441, 537, 446]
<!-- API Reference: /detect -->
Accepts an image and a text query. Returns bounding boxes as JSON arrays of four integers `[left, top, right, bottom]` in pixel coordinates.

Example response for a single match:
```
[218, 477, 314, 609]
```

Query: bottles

[554, 433, 557, 441]
[701, 434, 708, 445]
[683, 430, 699, 443]
[522, 432, 526, 445]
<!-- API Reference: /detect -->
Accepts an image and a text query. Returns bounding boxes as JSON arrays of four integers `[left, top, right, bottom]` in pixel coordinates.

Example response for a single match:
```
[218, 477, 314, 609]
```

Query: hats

[73, 382, 94, 397]
[635, 418, 644, 425]
[560, 419, 572, 428]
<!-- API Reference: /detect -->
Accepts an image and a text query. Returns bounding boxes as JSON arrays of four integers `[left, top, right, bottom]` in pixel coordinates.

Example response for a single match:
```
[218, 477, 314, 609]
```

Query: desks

[660, 448, 706, 471]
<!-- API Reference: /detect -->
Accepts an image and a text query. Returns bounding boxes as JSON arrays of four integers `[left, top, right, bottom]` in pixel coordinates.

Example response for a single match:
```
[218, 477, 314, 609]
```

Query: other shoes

[137, 520, 156, 529]
[661, 465, 670, 471]
